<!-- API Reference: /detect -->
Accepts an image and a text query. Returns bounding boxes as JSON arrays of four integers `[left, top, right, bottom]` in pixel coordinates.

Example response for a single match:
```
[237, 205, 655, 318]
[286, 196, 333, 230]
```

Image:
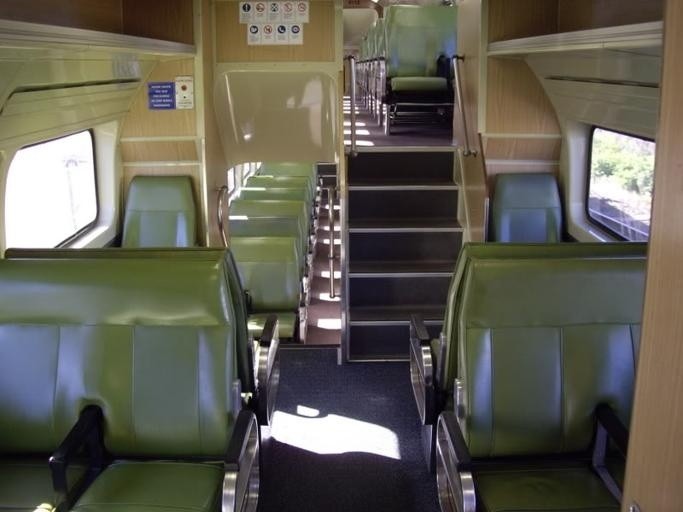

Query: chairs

[0, 159, 323, 512]
[408, 172, 648, 512]
[356, 4, 458, 137]
